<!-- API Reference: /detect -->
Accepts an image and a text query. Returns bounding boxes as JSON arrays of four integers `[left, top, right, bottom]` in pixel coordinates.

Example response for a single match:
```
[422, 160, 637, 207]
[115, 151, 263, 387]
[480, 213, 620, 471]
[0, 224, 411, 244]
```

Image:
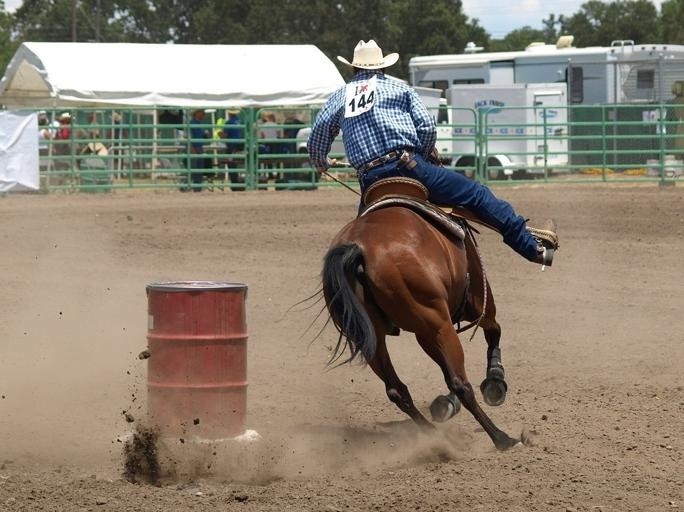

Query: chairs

[38, 157, 187, 195]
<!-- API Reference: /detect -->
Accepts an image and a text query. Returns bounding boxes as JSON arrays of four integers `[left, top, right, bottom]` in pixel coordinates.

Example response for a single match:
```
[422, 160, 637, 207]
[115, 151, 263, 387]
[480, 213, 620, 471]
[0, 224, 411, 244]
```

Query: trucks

[297, 84, 568, 180]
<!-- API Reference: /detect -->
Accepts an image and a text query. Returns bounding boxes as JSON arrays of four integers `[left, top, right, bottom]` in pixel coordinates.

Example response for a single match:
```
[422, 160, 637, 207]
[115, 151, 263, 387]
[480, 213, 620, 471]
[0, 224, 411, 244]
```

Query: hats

[337, 39, 400, 70]
[59, 112, 72, 121]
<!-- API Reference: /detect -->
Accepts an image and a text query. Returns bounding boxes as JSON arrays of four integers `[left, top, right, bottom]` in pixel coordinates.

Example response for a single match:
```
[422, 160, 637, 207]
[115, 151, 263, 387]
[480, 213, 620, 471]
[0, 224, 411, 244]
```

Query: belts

[357, 147, 409, 174]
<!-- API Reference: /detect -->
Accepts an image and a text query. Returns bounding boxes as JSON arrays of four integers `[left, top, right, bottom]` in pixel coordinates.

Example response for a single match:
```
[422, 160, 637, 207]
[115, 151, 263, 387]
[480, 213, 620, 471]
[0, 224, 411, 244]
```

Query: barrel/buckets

[147, 280, 248, 439]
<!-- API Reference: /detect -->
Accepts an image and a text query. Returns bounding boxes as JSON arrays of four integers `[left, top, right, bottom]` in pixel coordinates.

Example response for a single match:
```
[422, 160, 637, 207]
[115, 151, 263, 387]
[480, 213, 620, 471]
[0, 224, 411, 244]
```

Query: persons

[305, 38, 556, 268]
[37, 107, 320, 191]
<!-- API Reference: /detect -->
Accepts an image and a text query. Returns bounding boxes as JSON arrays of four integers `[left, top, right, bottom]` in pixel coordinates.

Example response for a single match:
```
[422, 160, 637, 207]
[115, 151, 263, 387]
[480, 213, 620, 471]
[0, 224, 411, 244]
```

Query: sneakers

[533, 218, 558, 266]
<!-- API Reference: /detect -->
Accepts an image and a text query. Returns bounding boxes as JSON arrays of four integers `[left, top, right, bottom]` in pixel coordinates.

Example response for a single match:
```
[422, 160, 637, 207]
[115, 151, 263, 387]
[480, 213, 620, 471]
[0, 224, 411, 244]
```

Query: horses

[283, 202, 525, 457]
